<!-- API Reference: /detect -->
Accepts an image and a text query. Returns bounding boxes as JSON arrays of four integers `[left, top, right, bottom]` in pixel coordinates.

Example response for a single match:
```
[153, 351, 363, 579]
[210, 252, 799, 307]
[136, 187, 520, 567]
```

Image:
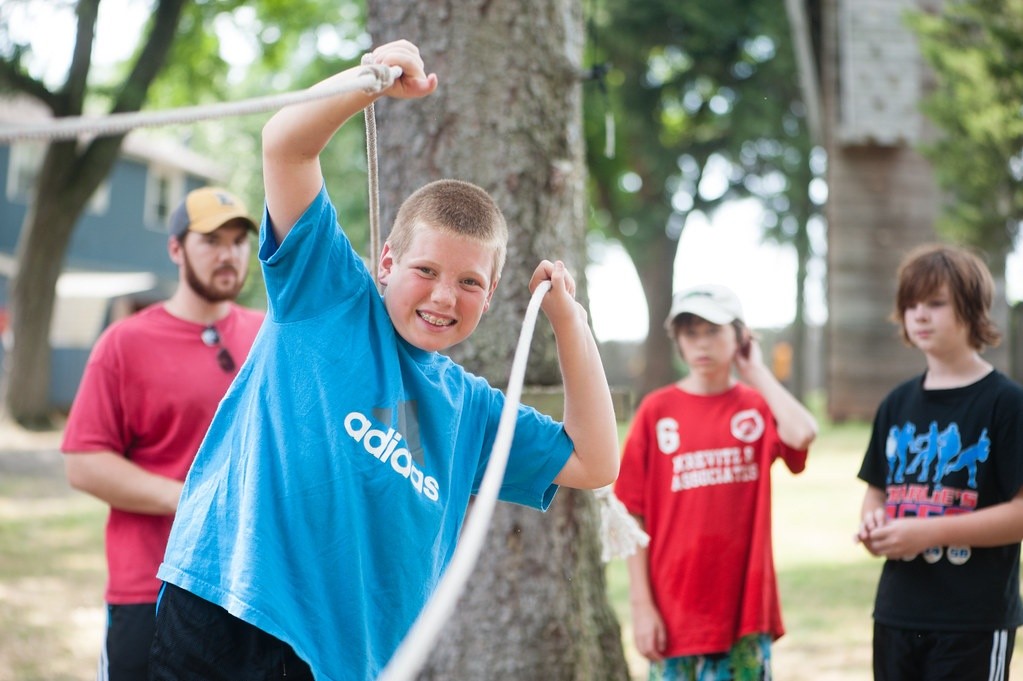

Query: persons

[611, 283, 818, 680]
[60, 186, 265, 680]
[851, 245, 1023, 681]
[145, 39, 622, 679]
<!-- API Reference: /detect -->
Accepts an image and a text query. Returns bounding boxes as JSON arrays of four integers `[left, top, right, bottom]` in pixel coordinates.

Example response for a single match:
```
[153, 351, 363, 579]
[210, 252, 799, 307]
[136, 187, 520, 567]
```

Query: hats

[664, 283, 747, 326]
[171, 186, 260, 236]
